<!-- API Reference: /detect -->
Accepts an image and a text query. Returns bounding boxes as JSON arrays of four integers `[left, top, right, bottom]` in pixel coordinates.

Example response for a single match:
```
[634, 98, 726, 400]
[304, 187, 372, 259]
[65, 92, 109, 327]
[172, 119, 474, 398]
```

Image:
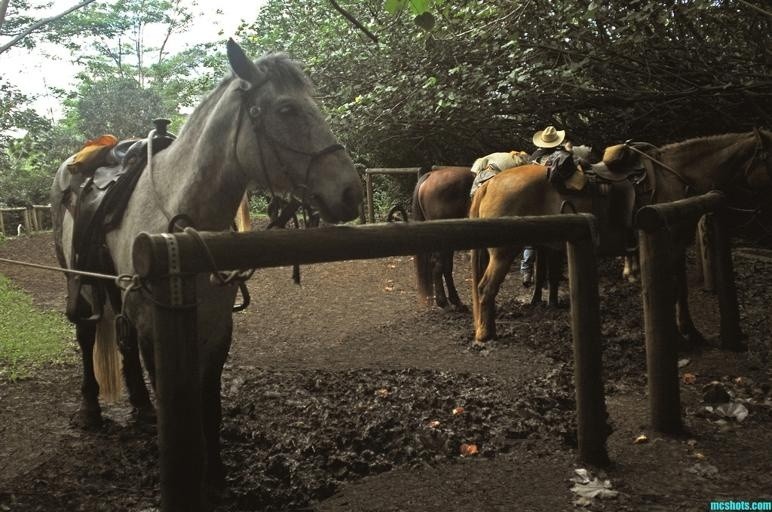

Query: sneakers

[523, 273, 531, 288]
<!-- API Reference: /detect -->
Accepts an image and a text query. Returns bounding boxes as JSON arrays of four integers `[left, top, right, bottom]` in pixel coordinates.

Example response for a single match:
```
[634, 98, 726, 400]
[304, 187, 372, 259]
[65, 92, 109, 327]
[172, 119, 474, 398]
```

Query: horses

[47, 35, 369, 494]
[408, 125, 772, 355]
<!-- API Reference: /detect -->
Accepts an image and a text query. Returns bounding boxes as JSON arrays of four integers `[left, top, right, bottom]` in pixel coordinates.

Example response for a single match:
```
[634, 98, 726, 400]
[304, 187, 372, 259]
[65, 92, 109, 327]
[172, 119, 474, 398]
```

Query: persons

[521, 127, 573, 286]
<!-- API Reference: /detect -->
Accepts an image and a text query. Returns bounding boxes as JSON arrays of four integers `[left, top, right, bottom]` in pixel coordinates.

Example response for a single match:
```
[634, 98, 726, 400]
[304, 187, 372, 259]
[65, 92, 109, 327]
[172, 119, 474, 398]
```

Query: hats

[533, 126, 566, 148]
